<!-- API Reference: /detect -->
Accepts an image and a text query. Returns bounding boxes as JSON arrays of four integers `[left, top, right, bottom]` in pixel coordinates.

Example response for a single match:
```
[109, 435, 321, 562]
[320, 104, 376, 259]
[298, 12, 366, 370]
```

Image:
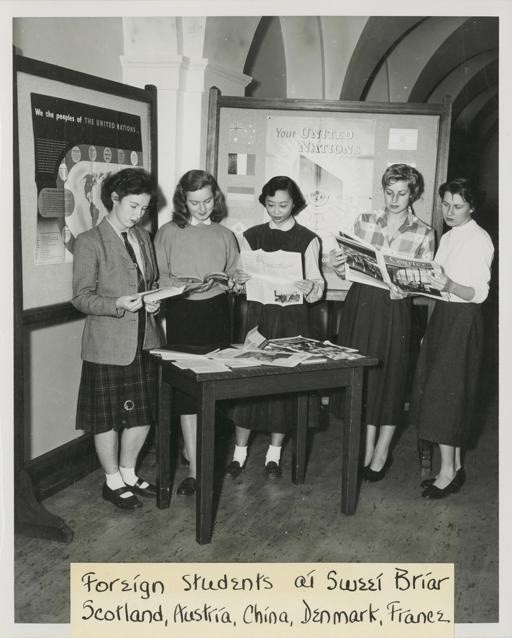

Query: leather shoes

[264, 461, 280, 481]
[421, 467, 466, 498]
[102, 480, 142, 509]
[222, 456, 248, 478]
[176, 477, 196, 497]
[358, 450, 393, 482]
[123, 477, 158, 497]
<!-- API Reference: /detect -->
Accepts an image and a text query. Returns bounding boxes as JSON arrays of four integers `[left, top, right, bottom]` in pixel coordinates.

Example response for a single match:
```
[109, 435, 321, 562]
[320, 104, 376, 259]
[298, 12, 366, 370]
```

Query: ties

[120, 232, 144, 333]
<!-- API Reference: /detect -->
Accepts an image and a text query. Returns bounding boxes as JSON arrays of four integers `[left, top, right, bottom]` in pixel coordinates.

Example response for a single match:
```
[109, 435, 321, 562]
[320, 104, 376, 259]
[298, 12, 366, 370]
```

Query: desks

[140, 338, 379, 545]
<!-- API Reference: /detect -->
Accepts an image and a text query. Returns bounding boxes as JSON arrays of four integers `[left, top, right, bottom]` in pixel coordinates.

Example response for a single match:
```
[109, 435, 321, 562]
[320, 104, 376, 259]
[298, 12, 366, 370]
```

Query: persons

[222, 175, 325, 481]
[69, 168, 165, 512]
[327, 164, 436, 483]
[389, 178, 495, 499]
[152, 170, 242, 498]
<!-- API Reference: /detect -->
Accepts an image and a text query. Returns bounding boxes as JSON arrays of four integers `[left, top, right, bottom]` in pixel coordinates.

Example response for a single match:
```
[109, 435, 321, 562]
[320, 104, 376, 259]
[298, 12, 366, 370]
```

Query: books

[169, 271, 228, 294]
[335, 229, 450, 301]
[132, 282, 201, 306]
